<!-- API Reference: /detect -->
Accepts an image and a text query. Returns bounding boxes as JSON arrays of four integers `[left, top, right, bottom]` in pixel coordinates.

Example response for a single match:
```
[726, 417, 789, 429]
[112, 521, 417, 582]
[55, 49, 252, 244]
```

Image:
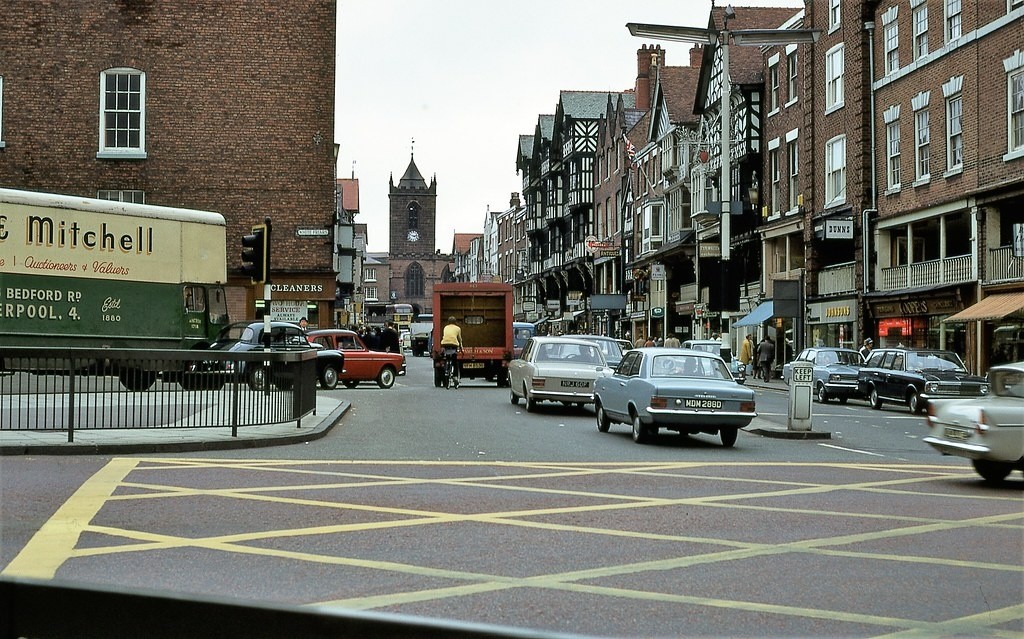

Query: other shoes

[455, 378, 458, 384]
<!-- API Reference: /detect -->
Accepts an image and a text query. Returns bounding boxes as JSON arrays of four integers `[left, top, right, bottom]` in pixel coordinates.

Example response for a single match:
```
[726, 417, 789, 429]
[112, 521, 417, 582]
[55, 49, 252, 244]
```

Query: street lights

[624, 4, 826, 375]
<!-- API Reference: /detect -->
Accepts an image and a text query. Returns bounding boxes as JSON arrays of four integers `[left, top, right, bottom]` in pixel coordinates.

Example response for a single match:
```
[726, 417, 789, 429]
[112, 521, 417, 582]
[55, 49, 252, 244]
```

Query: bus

[384, 304, 433, 358]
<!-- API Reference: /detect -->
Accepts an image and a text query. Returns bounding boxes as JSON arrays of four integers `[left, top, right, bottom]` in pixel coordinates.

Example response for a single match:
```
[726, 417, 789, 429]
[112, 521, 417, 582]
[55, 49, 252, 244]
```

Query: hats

[299, 317, 308, 323]
[866, 338, 873, 343]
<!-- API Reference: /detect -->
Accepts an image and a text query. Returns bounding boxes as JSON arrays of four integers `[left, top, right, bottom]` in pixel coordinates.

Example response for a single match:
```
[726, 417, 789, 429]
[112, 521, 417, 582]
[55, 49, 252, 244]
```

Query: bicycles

[441, 349, 464, 390]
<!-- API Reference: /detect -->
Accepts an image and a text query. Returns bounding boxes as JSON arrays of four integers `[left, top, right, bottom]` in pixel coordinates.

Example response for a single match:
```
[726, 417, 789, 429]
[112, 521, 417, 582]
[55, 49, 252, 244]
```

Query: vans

[512, 322, 534, 357]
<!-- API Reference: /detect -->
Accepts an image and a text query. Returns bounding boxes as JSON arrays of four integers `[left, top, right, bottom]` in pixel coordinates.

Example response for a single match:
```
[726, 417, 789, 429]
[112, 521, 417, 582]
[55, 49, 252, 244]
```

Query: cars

[855, 348, 992, 415]
[671, 340, 747, 385]
[781, 347, 866, 404]
[923, 361, 1024, 485]
[189, 320, 346, 392]
[307, 329, 407, 391]
[507, 336, 615, 412]
[590, 347, 758, 448]
[550, 334, 636, 370]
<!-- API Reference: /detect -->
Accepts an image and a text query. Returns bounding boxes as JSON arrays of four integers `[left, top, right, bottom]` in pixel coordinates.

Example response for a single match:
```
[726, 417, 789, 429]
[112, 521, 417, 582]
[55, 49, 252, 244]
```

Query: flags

[625, 138, 635, 161]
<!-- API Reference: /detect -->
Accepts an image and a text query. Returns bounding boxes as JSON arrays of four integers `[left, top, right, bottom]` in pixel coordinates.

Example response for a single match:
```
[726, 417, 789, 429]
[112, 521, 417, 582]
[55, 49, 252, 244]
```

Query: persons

[578, 345, 591, 356]
[441, 316, 464, 386]
[706, 333, 718, 352]
[291, 316, 308, 342]
[346, 320, 399, 353]
[859, 338, 873, 363]
[633, 333, 681, 348]
[740, 333, 774, 383]
[684, 358, 696, 373]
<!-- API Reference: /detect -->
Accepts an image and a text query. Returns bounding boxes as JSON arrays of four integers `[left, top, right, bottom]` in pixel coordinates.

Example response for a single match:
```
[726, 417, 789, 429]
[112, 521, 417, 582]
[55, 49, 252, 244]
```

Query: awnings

[730, 300, 773, 328]
[547, 310, 585, 322]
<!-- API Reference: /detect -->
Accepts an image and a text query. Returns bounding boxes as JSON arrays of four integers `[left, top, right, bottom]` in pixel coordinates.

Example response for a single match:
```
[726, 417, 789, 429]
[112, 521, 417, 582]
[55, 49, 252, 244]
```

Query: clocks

[406, 229, 420, 242]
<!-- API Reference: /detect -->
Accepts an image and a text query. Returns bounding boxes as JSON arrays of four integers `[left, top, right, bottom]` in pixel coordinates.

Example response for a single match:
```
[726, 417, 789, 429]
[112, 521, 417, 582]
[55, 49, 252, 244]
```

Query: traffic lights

[241, 224, 267, 286]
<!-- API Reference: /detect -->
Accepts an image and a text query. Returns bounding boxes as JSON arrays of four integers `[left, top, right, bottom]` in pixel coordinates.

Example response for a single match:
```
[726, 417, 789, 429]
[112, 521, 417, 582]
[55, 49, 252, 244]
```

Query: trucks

[0, 187, 230, 392]
[433, 282, 513, 388]
